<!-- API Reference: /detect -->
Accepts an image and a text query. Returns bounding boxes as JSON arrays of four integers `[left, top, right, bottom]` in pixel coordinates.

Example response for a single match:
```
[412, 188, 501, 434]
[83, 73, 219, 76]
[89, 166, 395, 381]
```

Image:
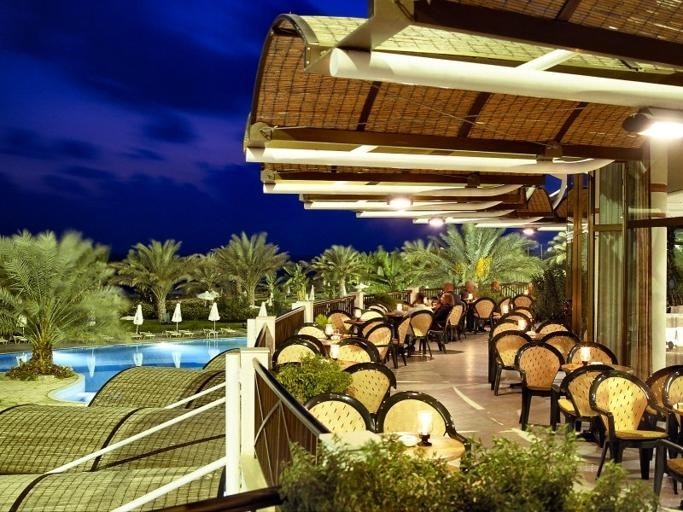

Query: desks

[375, 432, 465, 462]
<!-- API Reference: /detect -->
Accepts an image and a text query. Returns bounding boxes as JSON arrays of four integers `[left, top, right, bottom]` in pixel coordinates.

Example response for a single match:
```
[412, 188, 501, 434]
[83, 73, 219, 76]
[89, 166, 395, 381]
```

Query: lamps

[416, 410, 433, 446]
[428, 216, 446, 228]
[523, 227, 537, 235]
[387, 197, 413, 209]
[622, 107, 683, 141]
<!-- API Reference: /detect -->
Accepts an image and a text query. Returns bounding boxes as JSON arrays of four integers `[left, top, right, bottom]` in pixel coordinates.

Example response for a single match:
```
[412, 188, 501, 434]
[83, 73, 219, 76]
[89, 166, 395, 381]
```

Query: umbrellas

[171, 303, 183, 332]
[86, 302, 97, 342]
[16, 301, 27, 337]
[308, 285, 315, 302]
[338, 277, 347, 298]
[208, 302, 221, 331]
[268, 292, 274, 309]
[196, 290, 215, 308]
[133, 304, 144, 335]
[258, 301, 267, 316]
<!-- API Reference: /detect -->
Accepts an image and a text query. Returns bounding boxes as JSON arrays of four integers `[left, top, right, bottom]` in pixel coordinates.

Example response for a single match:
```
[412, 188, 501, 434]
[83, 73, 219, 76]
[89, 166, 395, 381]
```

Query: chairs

[645, 365, 682, 408]
[303, 393, 376, 434]
[662, 369, 683, 409]
[0, 335, 30, 344]
[376, 391, 472, 450]
[126, 326, 236, 341]
[271, 291, 497, 372]
[342, 363, 396, 422]
[487, 293, 619, 436]
[589, 370, 677, 496]
[653, 439, 683, 512]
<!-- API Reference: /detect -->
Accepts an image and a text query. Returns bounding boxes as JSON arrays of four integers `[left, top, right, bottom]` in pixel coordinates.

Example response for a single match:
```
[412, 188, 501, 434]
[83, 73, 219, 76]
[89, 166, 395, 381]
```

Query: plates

[400, 435, 418, 446]
